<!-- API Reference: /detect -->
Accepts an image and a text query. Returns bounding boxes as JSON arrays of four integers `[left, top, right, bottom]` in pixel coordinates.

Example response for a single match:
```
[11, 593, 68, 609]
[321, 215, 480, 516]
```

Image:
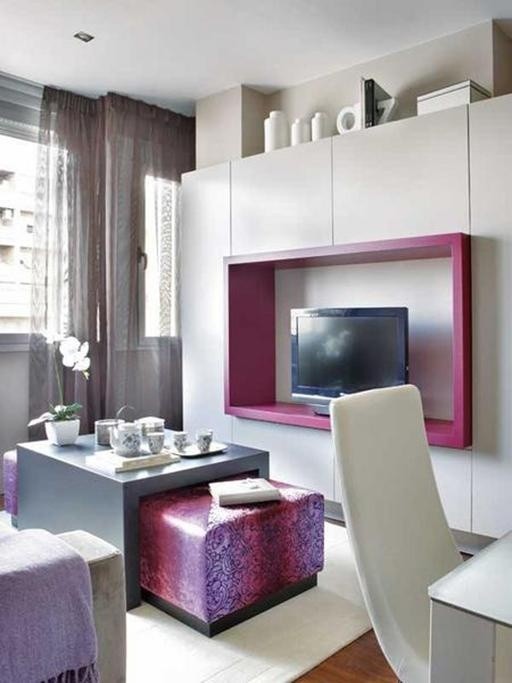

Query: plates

[170, 443, 228, 456]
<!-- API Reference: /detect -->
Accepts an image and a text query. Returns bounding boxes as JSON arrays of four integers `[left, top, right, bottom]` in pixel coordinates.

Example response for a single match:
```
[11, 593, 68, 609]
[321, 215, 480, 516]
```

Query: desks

[424, 530, 509, 682]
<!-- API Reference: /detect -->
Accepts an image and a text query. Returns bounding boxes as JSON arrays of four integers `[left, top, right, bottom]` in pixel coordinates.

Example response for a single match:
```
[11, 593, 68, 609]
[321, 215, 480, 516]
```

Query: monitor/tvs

[290, 307, 409, 416]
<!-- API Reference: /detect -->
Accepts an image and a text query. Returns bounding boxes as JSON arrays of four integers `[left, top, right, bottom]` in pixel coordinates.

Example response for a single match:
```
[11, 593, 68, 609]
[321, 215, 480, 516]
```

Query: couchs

[0, 515, 129, 681]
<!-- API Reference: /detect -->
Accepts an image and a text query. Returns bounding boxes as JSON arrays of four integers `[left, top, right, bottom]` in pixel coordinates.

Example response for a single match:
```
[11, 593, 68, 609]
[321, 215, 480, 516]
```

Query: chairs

[327, 382, 466, 683]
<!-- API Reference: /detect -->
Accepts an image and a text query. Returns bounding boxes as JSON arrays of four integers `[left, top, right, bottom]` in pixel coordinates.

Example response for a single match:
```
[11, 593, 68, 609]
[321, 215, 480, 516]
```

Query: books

[85, 455, 180, 472]
[208, 477, 280, 506]
[95, 445, 170, 466]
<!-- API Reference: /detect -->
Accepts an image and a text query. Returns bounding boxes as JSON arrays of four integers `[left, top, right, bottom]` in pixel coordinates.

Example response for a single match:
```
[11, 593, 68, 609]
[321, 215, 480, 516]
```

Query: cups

[147, 432, 164, 453]
[135, 416, 164, 446]
[195, 433, 211, 452]
[174, 431, 188, 451]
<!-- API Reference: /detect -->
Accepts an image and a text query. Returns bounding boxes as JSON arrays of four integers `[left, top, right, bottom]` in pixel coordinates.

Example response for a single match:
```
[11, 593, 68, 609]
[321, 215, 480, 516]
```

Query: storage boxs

[416, 76, 491, 113]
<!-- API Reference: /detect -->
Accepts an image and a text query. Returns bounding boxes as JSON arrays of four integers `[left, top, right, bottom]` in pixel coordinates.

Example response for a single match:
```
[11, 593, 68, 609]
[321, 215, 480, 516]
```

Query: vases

[41, 414, 83, 445]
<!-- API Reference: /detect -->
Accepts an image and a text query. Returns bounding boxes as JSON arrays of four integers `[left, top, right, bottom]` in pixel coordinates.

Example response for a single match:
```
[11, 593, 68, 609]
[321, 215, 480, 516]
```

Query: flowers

[42, 332, 93, 420]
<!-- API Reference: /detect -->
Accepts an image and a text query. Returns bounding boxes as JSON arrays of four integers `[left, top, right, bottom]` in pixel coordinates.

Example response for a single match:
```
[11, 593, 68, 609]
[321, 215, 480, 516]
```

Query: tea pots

[107, 405, 142, 457]
